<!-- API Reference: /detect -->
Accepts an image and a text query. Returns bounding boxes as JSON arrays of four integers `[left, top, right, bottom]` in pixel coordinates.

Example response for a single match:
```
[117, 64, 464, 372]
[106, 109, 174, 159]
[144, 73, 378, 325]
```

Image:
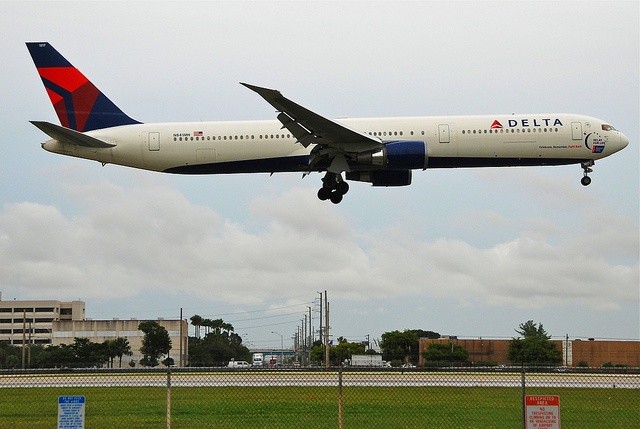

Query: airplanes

[25, 41, 629, 203]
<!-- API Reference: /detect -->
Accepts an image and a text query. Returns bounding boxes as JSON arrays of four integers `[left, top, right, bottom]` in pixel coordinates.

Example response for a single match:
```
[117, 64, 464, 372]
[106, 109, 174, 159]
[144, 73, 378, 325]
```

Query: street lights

[271, 332, 283, 363]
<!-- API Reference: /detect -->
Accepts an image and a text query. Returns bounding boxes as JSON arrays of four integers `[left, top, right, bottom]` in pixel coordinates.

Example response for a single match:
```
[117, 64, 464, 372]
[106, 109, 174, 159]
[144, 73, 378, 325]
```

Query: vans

[237, 361, 250, 367]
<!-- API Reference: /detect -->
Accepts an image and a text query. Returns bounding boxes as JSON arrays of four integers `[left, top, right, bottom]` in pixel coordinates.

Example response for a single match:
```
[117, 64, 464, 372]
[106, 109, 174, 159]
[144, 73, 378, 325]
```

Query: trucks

[253, 354, 263, 365]
[350, 355, 392, 367]
